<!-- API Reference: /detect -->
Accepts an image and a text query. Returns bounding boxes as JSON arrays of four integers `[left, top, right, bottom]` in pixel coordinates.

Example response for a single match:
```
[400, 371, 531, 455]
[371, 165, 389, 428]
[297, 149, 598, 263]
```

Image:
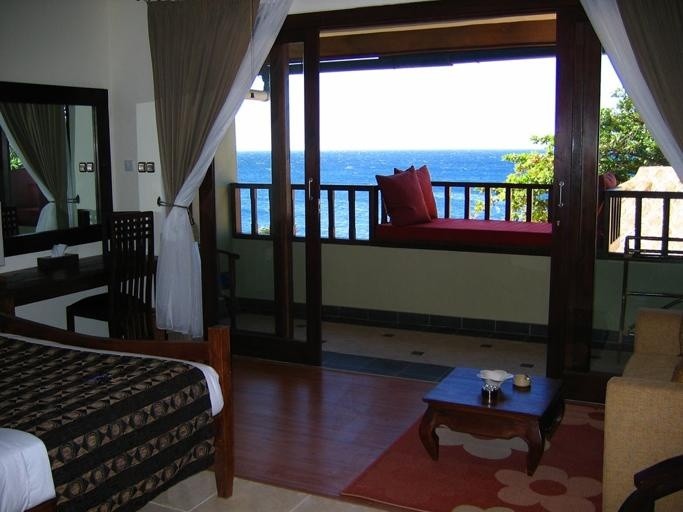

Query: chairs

[64, 209, 155, 343]
[194, 241, 240, 337]
[597, 307, 681, 511]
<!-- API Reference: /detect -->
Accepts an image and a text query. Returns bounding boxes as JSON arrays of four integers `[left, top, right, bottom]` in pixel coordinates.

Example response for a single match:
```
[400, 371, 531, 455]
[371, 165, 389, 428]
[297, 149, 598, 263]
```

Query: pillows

[393, 165, 438, 218]
[595, 170, 616, 229]
[376, 165, 432, 224]
[9, 165, 40, 210]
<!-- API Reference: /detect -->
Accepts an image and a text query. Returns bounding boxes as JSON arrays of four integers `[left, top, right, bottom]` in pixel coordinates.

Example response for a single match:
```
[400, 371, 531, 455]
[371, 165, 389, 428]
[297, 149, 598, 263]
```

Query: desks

[418, 365, 567, 479]
[0, 246, 157, 317]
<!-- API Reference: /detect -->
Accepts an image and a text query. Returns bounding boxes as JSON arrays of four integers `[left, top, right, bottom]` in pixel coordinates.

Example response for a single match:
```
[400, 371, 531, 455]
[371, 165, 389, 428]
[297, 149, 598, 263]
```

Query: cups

[480, 384, 498, 406]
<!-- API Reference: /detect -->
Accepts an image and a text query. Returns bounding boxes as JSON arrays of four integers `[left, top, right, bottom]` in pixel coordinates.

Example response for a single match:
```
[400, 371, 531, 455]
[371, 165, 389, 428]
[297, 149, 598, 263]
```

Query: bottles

[512, 372, 531, 387]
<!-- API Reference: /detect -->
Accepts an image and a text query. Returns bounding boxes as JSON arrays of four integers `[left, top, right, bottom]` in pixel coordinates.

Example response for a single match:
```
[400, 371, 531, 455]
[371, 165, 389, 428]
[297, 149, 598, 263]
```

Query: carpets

[342, 394, 605, 511]
[204, 350, 437, 500]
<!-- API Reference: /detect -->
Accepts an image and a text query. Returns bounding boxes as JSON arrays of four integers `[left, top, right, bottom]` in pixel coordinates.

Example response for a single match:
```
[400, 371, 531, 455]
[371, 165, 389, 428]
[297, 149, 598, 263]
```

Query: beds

[1, 306, 234, 510]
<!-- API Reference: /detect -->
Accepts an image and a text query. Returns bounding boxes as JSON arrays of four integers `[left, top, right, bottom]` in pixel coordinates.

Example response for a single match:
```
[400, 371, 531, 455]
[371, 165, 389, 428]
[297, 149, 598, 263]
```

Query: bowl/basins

[476, 369, 513, 389]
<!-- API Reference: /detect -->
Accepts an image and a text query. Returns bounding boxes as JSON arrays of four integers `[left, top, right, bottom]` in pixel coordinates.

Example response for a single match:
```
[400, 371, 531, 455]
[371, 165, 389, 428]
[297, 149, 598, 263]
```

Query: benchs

[373, 205, 555, 253]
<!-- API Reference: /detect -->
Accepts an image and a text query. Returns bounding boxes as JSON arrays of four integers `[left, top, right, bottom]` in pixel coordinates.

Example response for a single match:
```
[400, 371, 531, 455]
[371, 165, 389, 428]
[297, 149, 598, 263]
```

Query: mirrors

[0, 79, 113, 260]
